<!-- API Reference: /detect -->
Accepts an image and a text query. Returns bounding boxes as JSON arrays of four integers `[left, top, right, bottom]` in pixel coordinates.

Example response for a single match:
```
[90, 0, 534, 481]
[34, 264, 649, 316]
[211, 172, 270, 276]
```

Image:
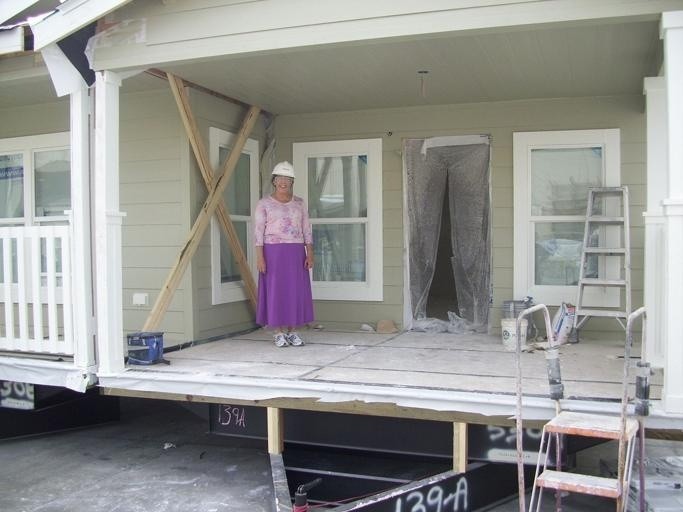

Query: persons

[252, 160, 314, 346]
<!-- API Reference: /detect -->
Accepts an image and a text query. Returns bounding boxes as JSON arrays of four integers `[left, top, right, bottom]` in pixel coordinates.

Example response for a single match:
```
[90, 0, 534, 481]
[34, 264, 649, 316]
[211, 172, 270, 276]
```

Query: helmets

[271, 160, 296, 179]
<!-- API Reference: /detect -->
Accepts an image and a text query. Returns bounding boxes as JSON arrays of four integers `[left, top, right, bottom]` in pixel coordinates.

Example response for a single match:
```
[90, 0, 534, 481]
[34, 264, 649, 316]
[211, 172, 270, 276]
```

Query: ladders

[569, 186, 633, 343]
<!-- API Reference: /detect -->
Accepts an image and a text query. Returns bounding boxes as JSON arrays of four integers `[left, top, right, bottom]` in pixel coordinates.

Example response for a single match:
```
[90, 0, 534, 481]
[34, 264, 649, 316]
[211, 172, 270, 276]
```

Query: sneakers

[273, 332, 288, 348]
[285, 333, 305, 346]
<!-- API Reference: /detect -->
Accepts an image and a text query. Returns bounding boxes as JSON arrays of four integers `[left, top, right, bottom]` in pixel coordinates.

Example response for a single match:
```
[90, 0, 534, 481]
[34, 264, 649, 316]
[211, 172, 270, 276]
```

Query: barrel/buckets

[502, 317, 528, 348]
